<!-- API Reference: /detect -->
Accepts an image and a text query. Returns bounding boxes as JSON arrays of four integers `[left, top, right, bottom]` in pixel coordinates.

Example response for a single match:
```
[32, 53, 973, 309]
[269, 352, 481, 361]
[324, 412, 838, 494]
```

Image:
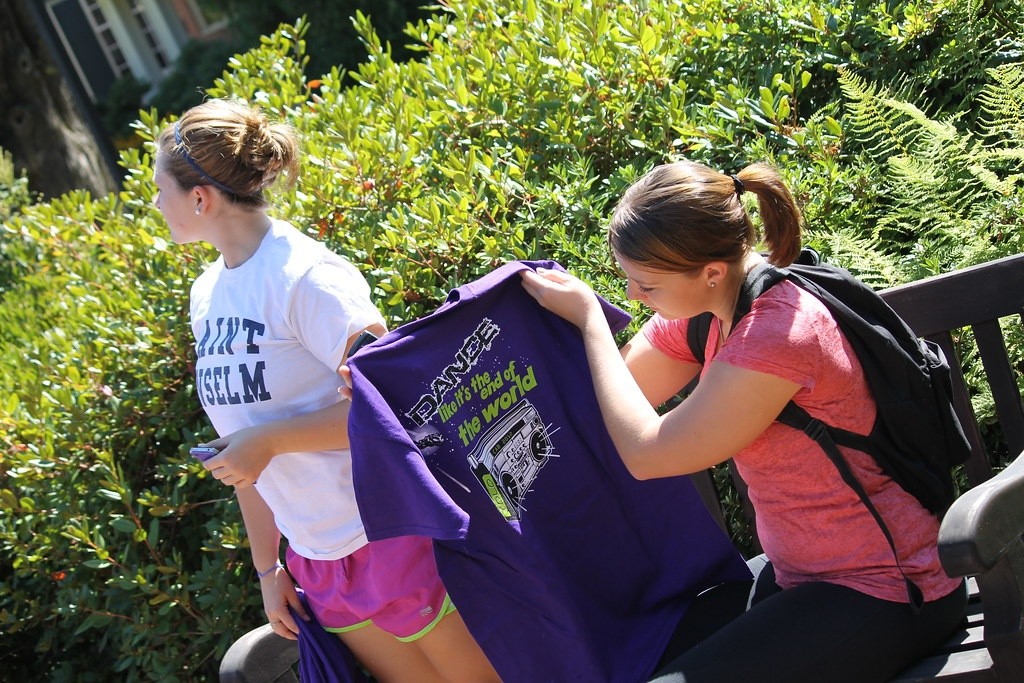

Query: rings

[271, 620, 282, 626]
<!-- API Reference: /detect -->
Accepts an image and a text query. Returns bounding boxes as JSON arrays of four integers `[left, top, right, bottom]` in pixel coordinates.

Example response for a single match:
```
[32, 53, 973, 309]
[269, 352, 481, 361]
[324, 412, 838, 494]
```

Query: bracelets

[255, 563, 284, 578]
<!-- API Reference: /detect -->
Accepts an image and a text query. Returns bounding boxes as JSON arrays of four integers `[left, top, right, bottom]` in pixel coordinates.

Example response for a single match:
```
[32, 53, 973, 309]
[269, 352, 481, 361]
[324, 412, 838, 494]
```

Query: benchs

[221, 251, 1024, 683]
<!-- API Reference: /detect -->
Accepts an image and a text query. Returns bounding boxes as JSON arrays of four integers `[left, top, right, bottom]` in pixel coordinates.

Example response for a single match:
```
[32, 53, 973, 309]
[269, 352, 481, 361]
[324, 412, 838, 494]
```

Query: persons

[149, 96, 504, 683]
[508, 158, 973, 682]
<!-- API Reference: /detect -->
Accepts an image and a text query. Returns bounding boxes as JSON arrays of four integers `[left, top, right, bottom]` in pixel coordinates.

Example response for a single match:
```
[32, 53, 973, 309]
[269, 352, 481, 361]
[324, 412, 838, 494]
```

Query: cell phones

[189, 448, 220, 462]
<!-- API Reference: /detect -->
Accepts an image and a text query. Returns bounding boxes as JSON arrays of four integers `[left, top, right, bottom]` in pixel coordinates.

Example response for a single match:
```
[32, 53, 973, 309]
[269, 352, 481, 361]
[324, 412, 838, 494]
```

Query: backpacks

[686, 246, 972, 524]
